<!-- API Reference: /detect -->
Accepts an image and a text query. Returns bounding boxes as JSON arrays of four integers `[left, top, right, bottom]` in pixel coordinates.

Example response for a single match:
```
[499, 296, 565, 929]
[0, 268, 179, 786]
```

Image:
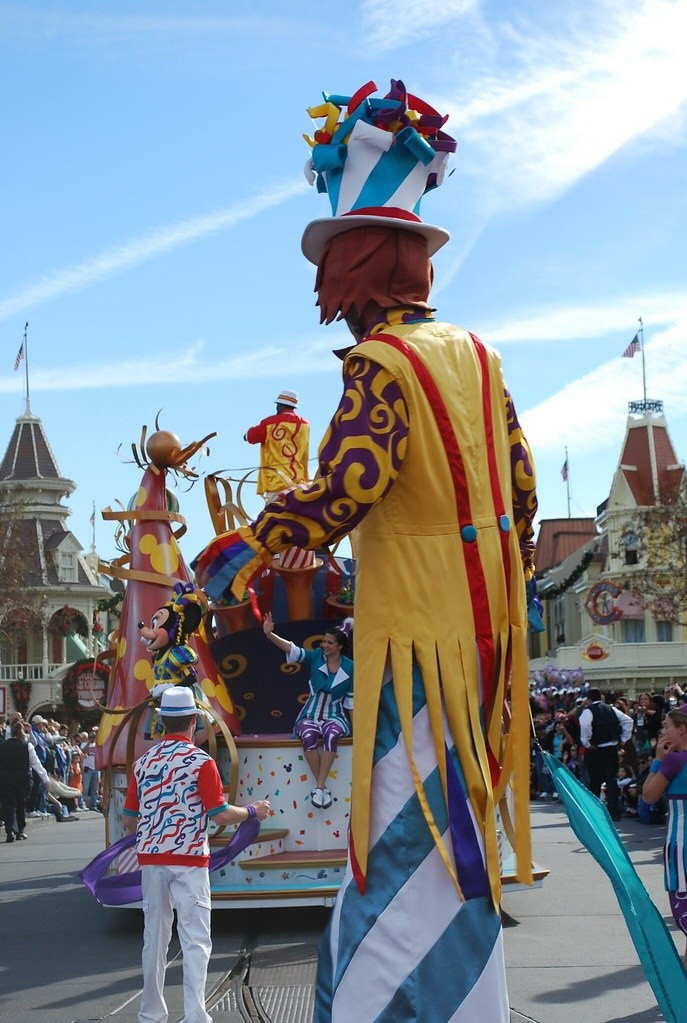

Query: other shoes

[6, 833, 15, 842]
[55, 816, 69, 822]
[551, 792, 559, 801]
[15, 833, 28, 840]
[73, 807, 82, 812]
[539, 792, 549, 800]
[24, 810, 51, 818]
[63, 815, 79, 821]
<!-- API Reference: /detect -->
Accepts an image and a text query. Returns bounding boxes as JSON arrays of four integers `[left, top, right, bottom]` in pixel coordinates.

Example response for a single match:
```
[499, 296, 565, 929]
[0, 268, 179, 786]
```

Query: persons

[243, 390, 310, 503]
[123, 686, 270, 1023]
[0, 713, 105, 842]
[530, 682, 687, 823]
[263, 611, 354, 809]
[641, 703, 687, 939]
[189, 229, 538, 969]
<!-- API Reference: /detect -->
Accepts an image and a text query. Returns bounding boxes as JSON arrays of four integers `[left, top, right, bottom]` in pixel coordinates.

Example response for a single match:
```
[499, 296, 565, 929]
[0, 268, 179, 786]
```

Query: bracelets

[650, 759, 662, 774]
[246, 805, 256, 820]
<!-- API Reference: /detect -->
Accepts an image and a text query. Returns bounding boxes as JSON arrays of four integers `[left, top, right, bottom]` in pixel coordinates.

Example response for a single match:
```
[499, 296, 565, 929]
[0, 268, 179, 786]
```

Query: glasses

[74, 740, 80, 742]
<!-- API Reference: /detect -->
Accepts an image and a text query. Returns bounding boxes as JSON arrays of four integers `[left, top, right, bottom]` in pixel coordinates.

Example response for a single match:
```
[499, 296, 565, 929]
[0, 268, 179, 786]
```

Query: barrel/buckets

[637, 794, 658, 825]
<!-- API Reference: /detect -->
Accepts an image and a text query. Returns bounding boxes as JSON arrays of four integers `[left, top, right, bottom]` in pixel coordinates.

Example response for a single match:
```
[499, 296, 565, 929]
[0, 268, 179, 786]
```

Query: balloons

[532, 666, 590, 708]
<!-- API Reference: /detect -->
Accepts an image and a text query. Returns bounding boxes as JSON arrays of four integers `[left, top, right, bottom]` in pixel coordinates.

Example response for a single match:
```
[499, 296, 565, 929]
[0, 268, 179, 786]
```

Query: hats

[301, 78, 458, 267]
[88, 731, 96, 738]
[154, 686, 202, 716]
[31, 714, 49, 725]
[273, 390, 298, 408]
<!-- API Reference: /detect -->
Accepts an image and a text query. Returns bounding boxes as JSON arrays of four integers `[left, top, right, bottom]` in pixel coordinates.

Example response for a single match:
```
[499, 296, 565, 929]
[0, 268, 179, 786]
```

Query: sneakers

[310, 785, 324, 808]
[323, 787, 333, 809]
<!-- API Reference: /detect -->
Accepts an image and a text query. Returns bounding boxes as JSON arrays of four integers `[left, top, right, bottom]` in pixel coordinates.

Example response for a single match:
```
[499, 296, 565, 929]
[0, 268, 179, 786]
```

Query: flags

[561, 461, 567, 480]
[15, 343, 24, 370]
[90, 511, 94, 526]
[623, 334, 641, 358]
